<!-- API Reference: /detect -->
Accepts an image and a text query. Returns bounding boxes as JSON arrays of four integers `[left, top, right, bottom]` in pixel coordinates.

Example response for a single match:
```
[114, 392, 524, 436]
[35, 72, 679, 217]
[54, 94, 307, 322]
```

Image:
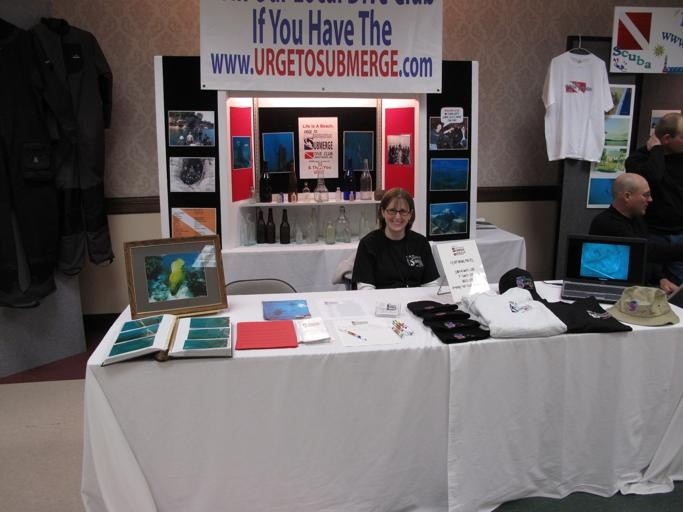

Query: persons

[588, 172, 679, 295]
[431, 121, 454, 150]
[176, 126, 212, 146]
[623, 112, 683, 307]
[351, 187, 442, 291]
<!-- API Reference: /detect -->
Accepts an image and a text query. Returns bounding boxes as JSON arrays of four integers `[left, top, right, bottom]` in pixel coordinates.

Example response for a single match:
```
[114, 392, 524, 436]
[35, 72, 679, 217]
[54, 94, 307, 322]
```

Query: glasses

[383, 208, 412, 216]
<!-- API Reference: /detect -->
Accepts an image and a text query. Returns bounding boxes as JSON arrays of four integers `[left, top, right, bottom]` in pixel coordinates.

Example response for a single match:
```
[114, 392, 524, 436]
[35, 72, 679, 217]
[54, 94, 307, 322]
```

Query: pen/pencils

[338, 328, 367, 341]
[391, 319, 414, 339]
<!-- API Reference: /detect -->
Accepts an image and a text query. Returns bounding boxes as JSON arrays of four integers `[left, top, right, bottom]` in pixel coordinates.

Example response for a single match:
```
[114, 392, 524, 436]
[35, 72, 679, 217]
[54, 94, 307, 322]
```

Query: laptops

[561, 233, 648, 303]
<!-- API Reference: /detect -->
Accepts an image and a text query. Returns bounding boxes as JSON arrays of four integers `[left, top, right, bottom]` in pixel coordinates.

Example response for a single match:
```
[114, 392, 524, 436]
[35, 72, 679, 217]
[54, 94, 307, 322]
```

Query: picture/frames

[123, 234, 228, 319]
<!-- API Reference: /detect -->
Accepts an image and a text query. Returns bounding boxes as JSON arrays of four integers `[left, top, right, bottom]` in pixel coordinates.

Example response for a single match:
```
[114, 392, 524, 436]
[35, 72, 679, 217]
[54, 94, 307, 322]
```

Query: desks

[82, 282, 683, 511]
[221, 218, 528, 293]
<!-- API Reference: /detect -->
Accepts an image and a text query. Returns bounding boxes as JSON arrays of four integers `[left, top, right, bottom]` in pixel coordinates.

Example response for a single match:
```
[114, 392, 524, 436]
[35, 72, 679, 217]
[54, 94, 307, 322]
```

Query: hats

[499, 267, 542, 302]
[603, 285, 680, 327]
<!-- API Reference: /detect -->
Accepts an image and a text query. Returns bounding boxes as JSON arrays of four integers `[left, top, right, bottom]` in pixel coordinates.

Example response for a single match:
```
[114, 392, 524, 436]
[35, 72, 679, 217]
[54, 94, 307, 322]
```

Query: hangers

[566, 32, 592, 55]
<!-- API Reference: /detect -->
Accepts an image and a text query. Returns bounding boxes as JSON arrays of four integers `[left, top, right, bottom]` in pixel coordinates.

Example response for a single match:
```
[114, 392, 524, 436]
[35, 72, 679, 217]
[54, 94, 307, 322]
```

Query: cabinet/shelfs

[154, 54, 479, 248]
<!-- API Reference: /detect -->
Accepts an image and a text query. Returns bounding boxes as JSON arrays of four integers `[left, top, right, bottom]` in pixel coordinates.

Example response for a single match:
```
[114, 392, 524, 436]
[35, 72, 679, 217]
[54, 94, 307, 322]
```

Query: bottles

[335, 187, 342, 201]
[257, 210, 266, 243]
[311, 208, 319, 241]
[288, 167, 298, 202]
[307, 217, 315, 243]
[296, 229, 303, 244]
[266, 208, 275, 243]
[344, 221, 351, 243]
[344, 152, 355, 200]
[349, 191, 354, 201]
[360, 159, 372, 200]
[365, 221, 370, 234]
[359, 206, 367, 240]
[303, 182, 310, 202]
[280, 192, 284, 202]
[280, 209, 290, 244]
[335, 206, 349, 242]
[314, 169, 329, 202]
[259, 161, 272, 203]
[244, 212, 256, 245]
[326, 218, 336, 244]
[278, 195, 282, 203]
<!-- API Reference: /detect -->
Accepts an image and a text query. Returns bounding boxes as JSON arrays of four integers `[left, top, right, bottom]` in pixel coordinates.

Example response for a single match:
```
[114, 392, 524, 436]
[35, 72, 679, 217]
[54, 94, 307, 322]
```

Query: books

[100, 312, 234, 368]
